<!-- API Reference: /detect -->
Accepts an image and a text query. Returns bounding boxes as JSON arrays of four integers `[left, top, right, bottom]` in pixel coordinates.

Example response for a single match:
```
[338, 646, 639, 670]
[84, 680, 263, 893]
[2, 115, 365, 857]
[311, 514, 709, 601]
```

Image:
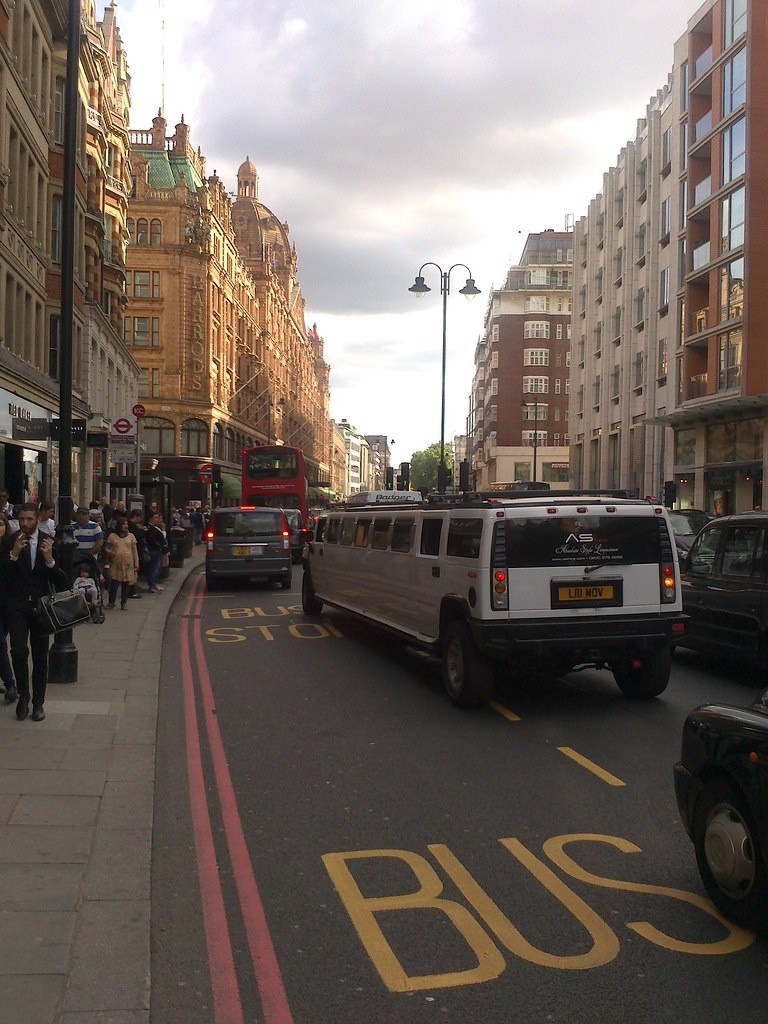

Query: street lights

[409, 261, 482, 495]
[518, 390, 541, 484]
[383, 437, 396, 491]
[268, 395, 288, 450]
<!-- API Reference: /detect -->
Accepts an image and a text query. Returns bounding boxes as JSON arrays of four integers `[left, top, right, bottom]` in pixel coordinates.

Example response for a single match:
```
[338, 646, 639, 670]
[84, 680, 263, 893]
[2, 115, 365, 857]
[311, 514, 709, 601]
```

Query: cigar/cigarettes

[19, 533, 23, 539]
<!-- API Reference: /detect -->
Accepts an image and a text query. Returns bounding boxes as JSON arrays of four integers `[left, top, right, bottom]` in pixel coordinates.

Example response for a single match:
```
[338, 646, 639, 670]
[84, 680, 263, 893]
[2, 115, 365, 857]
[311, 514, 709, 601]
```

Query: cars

[283, 507, 306, 565]
[671, 684, 767, 931]
[674, 509, 768, 682]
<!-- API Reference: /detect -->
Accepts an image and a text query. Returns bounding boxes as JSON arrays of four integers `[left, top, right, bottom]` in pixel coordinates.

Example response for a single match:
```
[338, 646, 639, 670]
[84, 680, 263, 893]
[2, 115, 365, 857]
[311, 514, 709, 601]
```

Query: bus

[240, 445, 311, 534]
[489, 480, 552, 494]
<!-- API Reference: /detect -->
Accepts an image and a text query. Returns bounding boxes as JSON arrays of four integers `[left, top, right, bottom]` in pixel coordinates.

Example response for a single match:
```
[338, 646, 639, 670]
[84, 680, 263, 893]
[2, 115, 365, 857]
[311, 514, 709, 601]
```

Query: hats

[77, 506, 89, 514]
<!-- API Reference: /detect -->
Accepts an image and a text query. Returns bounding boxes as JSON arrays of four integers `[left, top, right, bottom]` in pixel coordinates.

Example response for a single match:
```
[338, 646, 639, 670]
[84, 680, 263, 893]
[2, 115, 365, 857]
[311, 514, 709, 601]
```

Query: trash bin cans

[181, 526, 193, 559]
[168, 526, 186, 568]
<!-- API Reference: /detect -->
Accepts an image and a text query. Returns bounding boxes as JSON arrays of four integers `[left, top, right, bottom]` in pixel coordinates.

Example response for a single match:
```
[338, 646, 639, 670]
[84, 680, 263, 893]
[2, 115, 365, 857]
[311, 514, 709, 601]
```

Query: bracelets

[10, 551, 16, 560]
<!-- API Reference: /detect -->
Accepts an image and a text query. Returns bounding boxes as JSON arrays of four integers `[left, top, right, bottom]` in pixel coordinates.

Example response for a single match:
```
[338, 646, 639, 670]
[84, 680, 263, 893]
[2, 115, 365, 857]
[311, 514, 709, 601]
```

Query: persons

[0, 491, 222, 722]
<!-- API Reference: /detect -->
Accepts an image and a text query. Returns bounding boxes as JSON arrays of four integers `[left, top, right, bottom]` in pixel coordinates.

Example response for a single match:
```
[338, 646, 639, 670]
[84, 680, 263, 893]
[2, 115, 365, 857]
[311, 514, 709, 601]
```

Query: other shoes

[104, 604, 128, 610]
[128, 594, 142, 598]
[148, 586, 162, 594]
[156, 585, 164, 590]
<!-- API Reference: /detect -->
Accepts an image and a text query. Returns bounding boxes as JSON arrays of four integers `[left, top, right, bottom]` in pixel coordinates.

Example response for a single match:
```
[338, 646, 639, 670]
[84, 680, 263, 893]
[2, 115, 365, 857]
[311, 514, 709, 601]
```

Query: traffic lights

[385, 467, 393, 492]
[664, 479, 676, 509]
[439, 467, 452, 485]
[397, 475, 402, 491]
[402, 463, 410, 491]
[213, 467, 221, 491]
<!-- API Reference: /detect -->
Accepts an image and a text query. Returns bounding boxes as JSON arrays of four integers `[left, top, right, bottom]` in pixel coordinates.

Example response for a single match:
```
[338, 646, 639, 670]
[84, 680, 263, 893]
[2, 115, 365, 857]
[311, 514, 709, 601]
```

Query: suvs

[300, 485, 691, 708]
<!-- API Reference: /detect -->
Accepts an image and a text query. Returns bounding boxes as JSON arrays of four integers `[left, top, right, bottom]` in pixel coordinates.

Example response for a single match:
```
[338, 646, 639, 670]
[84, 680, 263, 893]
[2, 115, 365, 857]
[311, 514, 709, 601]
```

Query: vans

[203, 506, 293, 590]
[344, 491, 423, 509]
[665, 508, 720, 572]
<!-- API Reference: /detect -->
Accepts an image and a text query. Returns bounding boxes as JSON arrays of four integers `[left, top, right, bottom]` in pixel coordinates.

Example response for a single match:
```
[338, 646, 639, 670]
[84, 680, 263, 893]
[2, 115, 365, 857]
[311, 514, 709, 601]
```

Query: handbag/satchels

[162, 545, 169, 554]
[33, 588, 92, 636]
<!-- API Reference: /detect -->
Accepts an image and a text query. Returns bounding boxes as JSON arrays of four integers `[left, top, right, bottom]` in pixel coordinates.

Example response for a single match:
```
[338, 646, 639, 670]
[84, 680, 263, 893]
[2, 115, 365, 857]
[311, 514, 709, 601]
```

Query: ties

[25, 537, 32, 577]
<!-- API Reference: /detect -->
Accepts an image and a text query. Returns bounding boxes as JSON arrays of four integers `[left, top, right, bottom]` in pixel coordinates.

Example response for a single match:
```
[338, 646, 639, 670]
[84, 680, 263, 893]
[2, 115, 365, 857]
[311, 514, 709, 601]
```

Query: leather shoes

[16, 693, 31, 722]
[31, 704, 46, 721]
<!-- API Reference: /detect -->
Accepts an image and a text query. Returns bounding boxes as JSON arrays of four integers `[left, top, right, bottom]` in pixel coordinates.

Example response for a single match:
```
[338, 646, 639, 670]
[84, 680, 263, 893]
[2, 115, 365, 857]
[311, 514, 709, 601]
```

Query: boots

[3, 679, 18, 702]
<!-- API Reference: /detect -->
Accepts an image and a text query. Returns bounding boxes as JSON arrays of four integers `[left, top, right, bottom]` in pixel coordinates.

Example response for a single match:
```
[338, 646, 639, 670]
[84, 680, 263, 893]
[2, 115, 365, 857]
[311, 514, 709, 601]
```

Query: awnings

[222, 473, 243, 499]
[309, 487, 330, 500]
